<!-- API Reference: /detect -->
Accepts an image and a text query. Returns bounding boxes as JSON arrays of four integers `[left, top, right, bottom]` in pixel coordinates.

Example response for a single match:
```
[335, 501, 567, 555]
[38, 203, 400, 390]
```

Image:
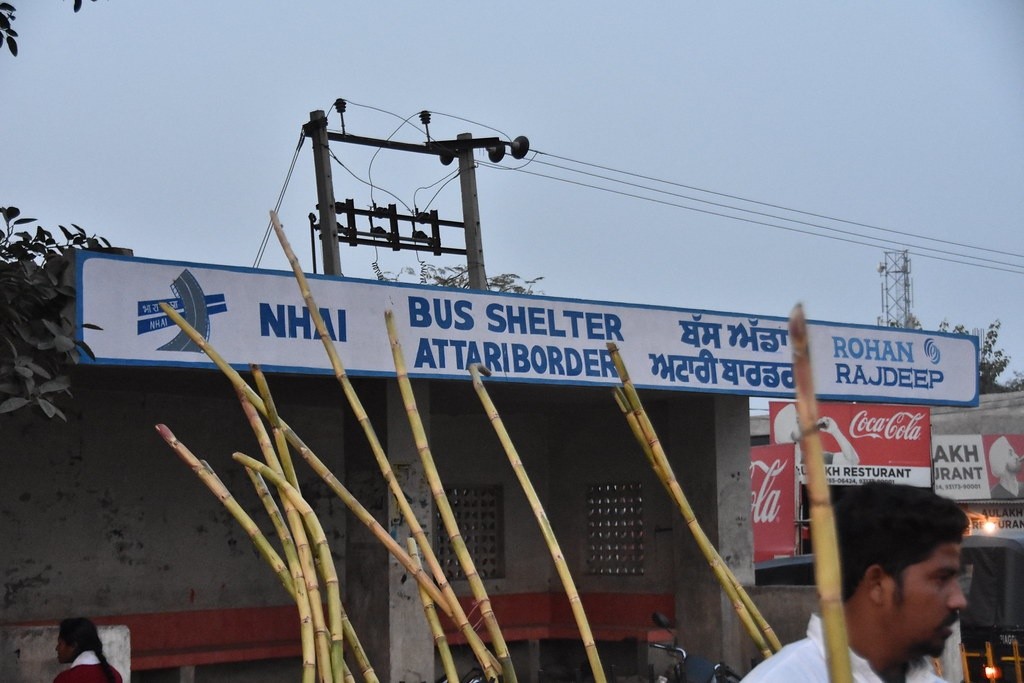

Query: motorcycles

[648, 613, 742, 683]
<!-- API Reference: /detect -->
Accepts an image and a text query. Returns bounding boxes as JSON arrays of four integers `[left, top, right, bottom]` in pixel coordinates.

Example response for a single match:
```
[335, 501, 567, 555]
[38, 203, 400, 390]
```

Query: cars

[962, 625, 1024, 666]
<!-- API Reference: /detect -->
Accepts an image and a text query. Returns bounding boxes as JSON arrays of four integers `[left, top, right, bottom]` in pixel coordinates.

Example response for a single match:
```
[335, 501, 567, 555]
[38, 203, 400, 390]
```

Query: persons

[741, 484, 969, 683]
[52, 617, 123, 683]
